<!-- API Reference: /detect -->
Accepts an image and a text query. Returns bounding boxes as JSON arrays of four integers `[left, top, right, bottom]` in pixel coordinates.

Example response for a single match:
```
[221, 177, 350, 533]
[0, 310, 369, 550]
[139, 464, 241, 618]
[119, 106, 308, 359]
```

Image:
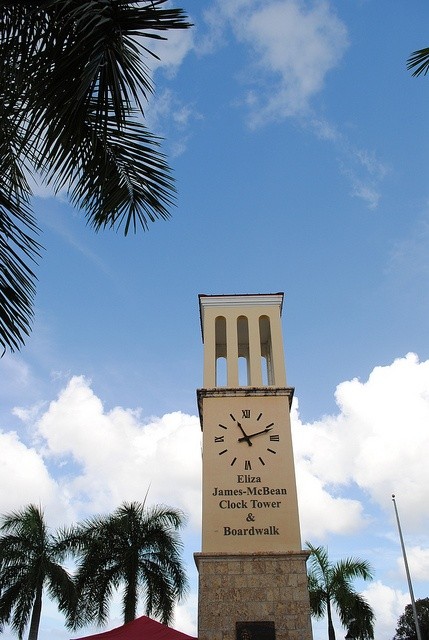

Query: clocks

[213, 407, 280, 472]
